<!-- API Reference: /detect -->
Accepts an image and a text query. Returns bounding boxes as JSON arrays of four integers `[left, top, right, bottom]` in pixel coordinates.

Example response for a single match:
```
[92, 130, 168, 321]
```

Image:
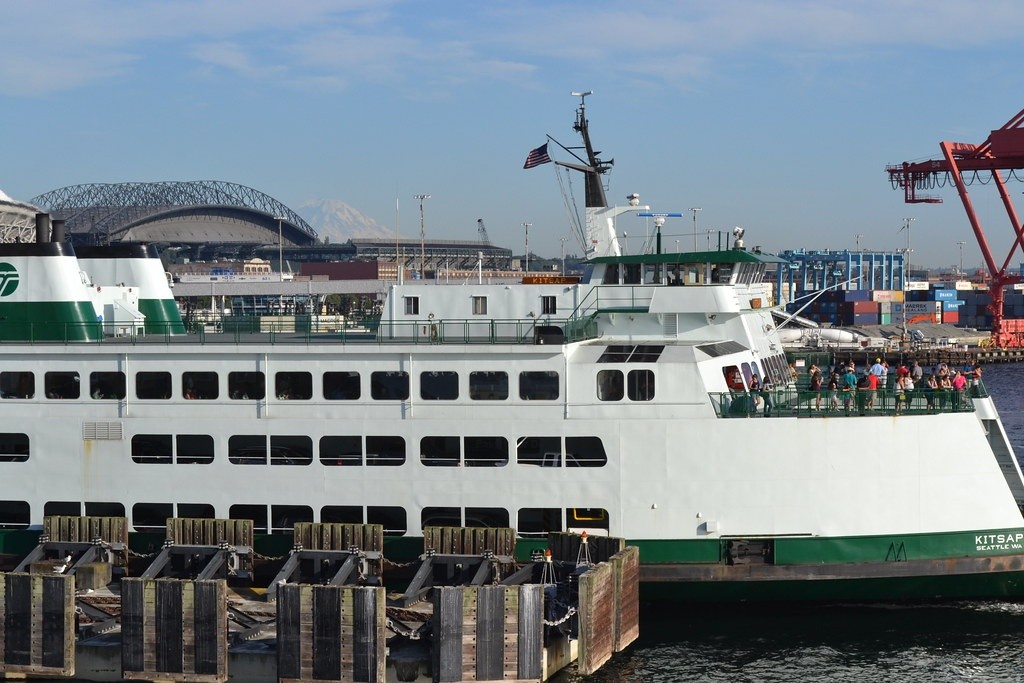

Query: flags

[523, 141, 554, 169]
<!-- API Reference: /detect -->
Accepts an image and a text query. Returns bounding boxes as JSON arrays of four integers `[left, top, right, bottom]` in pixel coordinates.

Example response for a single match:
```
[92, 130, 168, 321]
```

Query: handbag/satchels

[899, 394, 906, 400]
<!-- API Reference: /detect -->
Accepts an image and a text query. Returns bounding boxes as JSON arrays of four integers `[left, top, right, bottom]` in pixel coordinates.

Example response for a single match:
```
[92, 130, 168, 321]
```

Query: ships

[0, 90, 1024, 599]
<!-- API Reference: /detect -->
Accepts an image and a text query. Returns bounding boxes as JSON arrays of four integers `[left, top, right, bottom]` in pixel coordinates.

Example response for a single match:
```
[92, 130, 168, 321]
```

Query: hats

[875, 357, 881, 363]
[846, 367, 855, 371]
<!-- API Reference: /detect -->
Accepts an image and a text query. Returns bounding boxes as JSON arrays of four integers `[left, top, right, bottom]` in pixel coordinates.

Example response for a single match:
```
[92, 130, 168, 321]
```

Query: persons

[825, 356, 983, 399]
[952, 369, 967, 410]
[924, 374, 938, 414]
[749, 372, 761, 411]
[789, 362, 801, 396]
[807, 363, 821, 388]
[842, 367, 858, 416]
[811, 370, 824, 413]
[828, 374, 840, 412]
[893, 374, 908, 415]
[856, 371, 872, 415]
[827, 372, 835, 412]
[760, 376, 775, 416]
[903, 371, 915, 415]
[939, 374, 953, 412]
[865, 371, 881, 415]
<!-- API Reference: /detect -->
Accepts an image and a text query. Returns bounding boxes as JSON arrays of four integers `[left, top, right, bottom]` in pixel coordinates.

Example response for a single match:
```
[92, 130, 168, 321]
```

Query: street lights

[560, 238, 568, 276]
[615, 207, 728, 256]
[956, 240, 967, 282]
[520, 222, 533, 272]
[274, 216, 287, 279]
[413, 194, 431, 278]
[902, 217, 916, 290]
[895, 248, 915, 340]
